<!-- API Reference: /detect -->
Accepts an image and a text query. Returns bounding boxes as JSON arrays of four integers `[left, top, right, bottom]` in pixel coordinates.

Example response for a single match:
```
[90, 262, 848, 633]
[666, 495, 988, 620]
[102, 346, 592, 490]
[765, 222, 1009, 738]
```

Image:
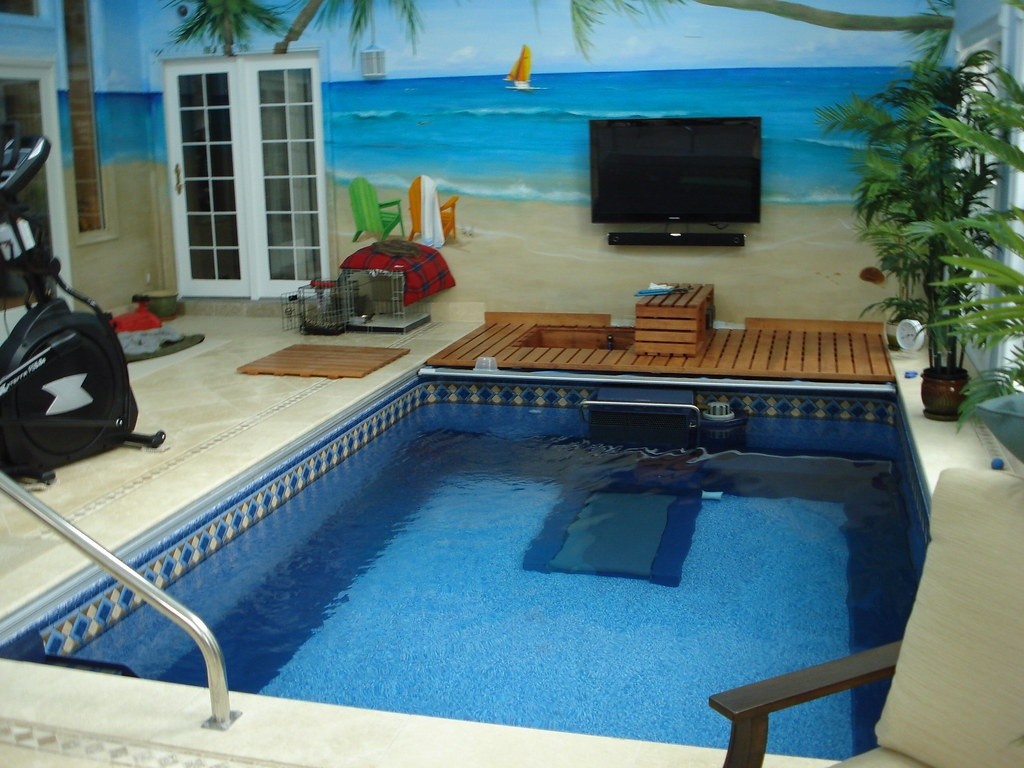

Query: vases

[143, 288, 179, 321]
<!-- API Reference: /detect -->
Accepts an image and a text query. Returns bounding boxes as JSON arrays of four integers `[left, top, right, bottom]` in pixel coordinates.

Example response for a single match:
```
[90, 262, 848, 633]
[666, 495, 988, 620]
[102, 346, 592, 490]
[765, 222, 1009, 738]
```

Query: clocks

[896, 320, 925, 351]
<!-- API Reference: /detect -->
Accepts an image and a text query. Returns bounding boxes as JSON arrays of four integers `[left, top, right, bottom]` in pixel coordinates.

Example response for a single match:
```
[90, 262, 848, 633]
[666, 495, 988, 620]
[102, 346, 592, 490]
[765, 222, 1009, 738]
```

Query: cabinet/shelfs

[635, 282, 715, 358]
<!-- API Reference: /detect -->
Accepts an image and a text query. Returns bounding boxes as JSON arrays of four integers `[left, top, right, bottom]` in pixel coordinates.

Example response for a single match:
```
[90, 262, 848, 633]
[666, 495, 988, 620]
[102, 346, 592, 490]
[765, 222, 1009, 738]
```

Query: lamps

[360, 47, 386, 77]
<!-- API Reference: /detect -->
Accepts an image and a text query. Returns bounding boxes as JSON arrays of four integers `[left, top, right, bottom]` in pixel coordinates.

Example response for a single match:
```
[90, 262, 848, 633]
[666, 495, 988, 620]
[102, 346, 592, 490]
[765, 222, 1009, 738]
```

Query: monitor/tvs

[590, 116, 762, 225]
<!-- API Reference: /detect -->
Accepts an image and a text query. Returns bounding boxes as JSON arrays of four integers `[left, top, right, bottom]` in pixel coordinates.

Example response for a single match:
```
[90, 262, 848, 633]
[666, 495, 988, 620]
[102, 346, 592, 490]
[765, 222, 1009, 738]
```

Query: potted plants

[853, 211, 923, 351]
[817, 51, 1023, 421]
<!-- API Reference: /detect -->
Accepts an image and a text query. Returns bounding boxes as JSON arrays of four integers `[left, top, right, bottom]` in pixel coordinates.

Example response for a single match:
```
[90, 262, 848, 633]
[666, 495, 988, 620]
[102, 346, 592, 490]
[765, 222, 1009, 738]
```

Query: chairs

[708, 465, 1024, 768]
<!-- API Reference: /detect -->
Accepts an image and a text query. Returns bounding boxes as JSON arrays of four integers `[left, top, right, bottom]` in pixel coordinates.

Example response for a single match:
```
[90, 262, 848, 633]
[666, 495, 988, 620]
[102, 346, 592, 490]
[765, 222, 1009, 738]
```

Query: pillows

[977, 395, 1024, 462]
